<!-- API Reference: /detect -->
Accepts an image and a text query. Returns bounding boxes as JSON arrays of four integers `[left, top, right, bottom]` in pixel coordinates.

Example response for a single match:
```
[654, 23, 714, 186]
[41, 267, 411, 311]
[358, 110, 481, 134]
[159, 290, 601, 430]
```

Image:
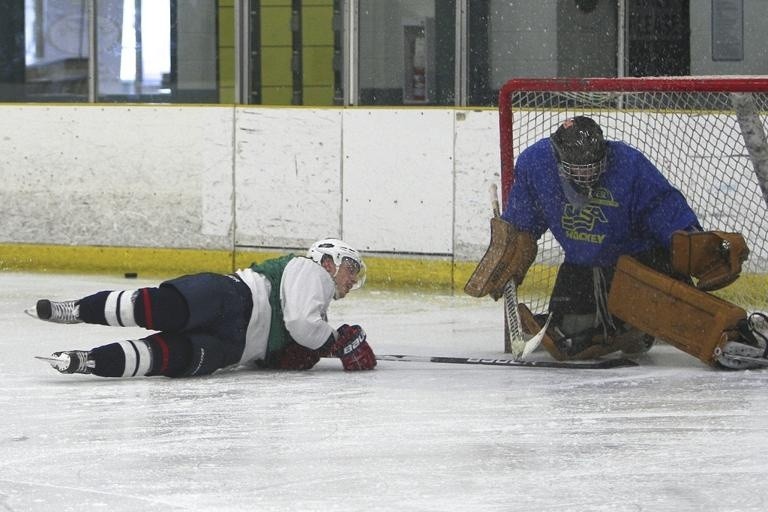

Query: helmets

[551, 115, 609, 189]
[306, 236, 367, 301]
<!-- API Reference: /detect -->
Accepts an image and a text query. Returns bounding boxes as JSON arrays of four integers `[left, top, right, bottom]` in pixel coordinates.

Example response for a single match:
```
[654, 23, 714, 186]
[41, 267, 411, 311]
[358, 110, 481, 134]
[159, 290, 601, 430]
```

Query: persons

[35, 237, 377, 380]
[462, 113, 767, 370]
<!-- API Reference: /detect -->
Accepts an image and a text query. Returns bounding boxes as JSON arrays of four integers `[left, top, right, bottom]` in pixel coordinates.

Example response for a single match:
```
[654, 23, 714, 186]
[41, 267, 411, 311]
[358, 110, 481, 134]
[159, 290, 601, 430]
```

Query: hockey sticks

[488, 184, 553, 362]
[375, 354, 636, 369]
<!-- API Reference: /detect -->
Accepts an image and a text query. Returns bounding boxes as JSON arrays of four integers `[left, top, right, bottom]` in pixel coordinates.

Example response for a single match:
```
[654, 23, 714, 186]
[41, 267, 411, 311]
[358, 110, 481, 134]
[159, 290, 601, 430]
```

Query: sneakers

[35, 298, 83, 325]
[48, 349, 96, 375]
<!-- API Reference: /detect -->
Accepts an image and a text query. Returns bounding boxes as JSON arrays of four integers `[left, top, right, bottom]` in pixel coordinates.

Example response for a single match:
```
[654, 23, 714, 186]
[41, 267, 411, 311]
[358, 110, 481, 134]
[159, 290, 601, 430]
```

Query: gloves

[335, 324, 376, 372]
